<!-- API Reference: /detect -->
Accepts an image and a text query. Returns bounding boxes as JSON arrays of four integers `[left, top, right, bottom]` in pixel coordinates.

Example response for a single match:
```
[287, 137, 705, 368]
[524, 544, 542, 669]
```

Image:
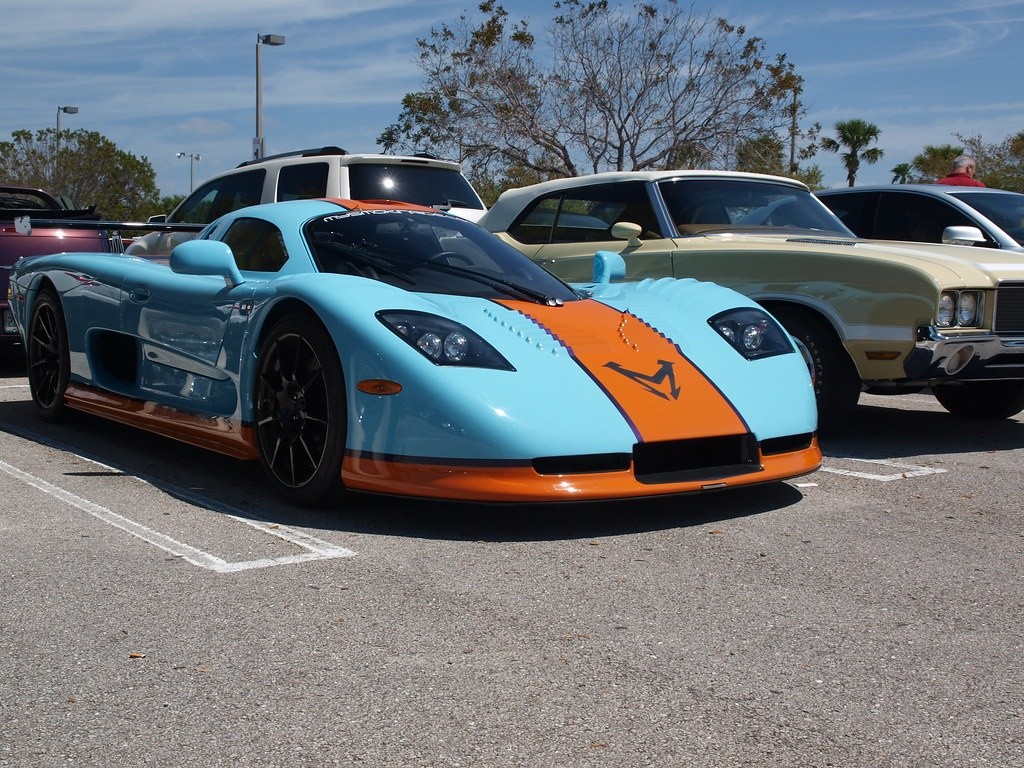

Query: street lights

[176, 152, 201, 195]
[256, 33, 286, 158]
[56, 106, 78, 167]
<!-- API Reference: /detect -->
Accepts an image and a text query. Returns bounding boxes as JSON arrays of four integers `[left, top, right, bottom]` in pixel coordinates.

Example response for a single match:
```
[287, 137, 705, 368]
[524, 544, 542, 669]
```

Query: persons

[933, 155, 985, 187]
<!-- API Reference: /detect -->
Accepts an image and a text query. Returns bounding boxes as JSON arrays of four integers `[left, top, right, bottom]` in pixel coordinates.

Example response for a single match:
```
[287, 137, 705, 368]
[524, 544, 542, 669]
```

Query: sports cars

[7, 199, 822, 503]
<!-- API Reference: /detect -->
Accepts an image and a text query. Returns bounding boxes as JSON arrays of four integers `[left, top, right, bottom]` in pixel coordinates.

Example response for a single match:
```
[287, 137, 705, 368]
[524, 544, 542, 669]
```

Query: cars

[731, 182, 1024, 249]
[0, 185, 110, 361]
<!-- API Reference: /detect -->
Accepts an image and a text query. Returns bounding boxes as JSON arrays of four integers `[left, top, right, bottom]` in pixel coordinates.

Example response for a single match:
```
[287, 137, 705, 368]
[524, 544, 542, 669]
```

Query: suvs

[478, 170, 1024, 421]
[126, 147, 490, 260]
